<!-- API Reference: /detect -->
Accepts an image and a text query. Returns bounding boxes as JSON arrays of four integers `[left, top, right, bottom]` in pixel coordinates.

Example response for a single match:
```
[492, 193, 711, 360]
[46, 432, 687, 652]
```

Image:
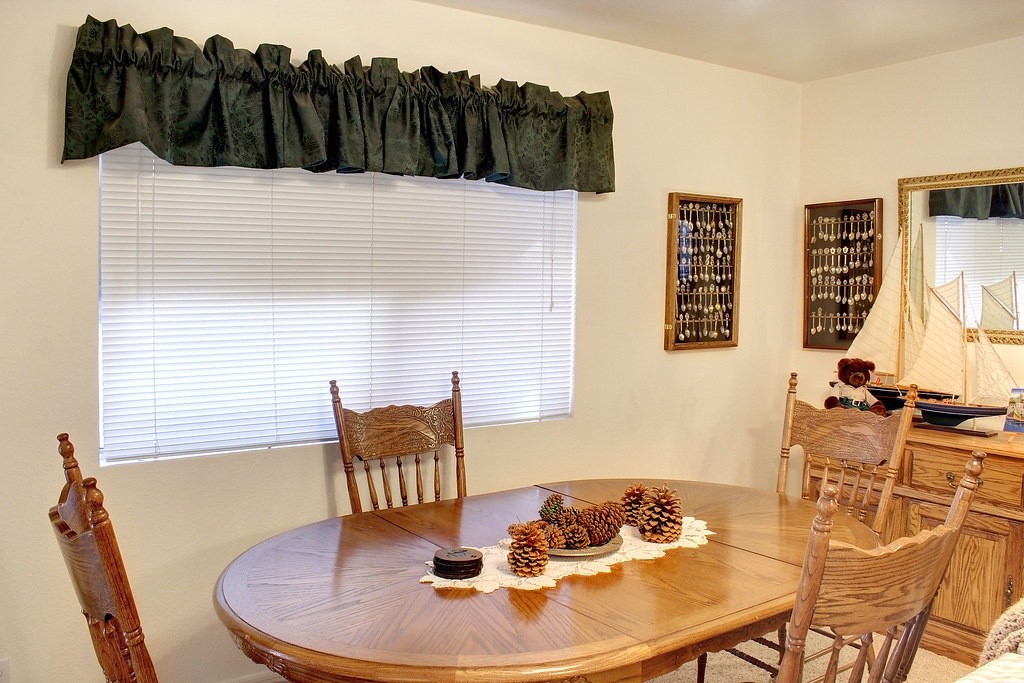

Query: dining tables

[211, 476, 884, 683]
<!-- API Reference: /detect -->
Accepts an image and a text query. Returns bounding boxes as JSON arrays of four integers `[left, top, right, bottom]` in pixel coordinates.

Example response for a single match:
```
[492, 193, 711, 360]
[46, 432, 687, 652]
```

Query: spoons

[810, 210, 874, 334]
[677, 202, 733, 340]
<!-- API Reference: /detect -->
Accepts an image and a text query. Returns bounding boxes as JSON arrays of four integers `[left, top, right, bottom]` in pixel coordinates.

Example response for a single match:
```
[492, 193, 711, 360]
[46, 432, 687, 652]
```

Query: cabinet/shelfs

[800, 439, 1023, 670]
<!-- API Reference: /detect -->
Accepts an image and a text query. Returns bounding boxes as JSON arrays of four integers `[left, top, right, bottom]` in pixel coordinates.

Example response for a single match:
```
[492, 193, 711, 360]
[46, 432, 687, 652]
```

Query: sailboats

[894, 273, 1021, 428]
[827, 221, 958, 420]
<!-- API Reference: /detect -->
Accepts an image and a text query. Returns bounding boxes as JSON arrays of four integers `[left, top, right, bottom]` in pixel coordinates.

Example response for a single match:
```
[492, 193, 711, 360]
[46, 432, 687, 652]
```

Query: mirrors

[895, 166, 1024, 409]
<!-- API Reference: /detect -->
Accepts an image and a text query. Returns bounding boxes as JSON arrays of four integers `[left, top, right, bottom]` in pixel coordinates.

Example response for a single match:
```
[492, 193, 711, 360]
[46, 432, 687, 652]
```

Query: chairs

[772, 451, 987, 683]
[44, 431, 161, 683]
[696, 370, 918, 683]
[328, 369, 469, 516]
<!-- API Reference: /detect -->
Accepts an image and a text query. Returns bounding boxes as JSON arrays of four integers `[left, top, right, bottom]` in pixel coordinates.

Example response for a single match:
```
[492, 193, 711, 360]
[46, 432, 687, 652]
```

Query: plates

[548, 534, 623, 557]
